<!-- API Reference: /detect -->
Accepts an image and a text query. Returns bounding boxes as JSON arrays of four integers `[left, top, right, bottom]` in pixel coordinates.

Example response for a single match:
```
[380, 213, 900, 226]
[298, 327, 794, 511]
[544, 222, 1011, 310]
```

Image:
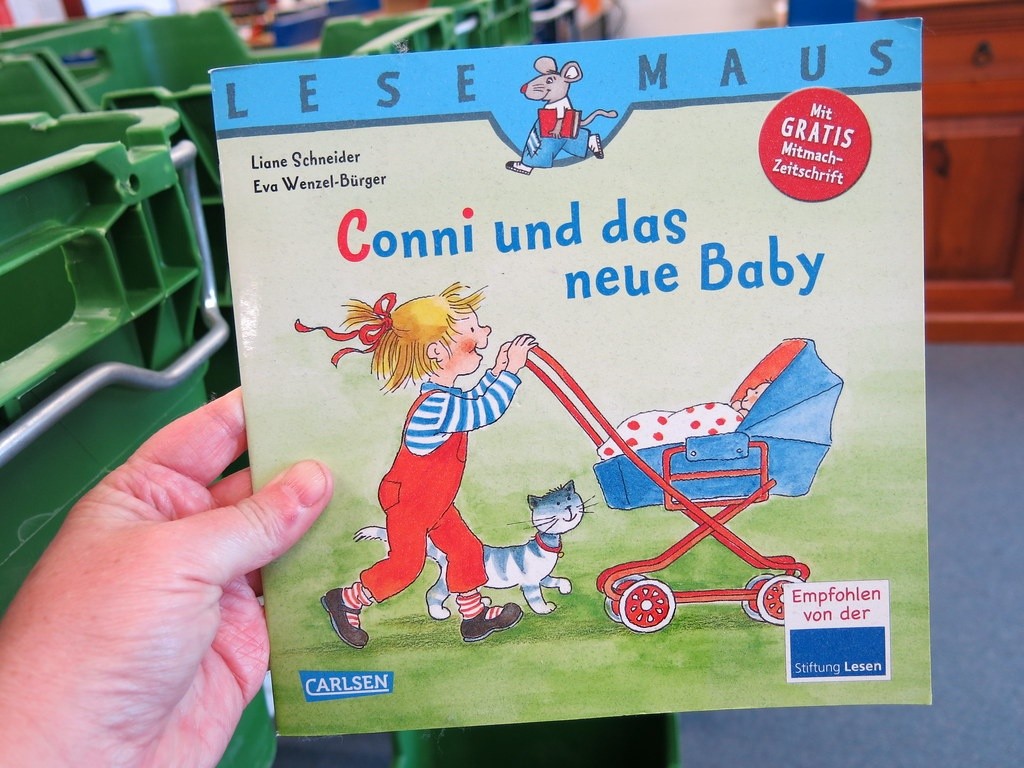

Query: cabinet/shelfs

[856, 0, 1024, 346]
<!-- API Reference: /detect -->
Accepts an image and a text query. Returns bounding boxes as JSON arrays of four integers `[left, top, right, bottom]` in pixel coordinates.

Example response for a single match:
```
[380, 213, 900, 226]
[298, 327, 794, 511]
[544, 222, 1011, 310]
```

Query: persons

[1, 385, 334, 767]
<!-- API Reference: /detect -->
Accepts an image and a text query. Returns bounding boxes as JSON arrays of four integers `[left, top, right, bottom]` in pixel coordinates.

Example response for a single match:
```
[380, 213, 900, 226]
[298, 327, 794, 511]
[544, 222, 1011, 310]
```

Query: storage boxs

[0, 0, 678, 768]
